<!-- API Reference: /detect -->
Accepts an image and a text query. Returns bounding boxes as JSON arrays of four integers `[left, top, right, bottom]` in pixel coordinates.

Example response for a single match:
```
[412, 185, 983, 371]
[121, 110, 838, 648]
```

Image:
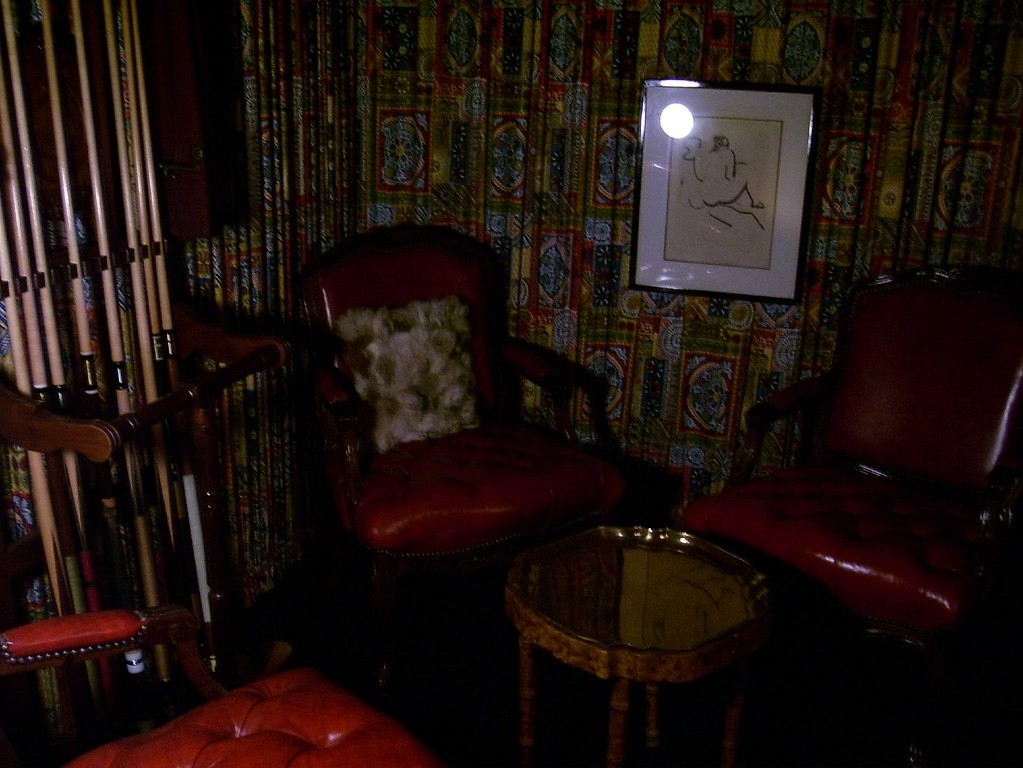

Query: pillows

[342, 293, 484, 455]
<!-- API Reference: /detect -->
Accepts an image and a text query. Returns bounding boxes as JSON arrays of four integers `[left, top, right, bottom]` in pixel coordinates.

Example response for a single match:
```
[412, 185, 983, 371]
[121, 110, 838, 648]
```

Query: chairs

[298, 222, 630, 721]
[0, 605, 442, 768]
[677, 263, 1023, 768]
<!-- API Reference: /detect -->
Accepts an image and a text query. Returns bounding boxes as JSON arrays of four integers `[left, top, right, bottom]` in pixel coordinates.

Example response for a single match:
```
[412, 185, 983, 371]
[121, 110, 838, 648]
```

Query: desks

[502, 522, 773, 768]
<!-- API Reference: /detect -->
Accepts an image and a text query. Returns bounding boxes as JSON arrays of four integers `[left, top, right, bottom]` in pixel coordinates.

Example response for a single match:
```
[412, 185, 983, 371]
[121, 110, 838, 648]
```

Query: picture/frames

[628, 78, 822, 306]
